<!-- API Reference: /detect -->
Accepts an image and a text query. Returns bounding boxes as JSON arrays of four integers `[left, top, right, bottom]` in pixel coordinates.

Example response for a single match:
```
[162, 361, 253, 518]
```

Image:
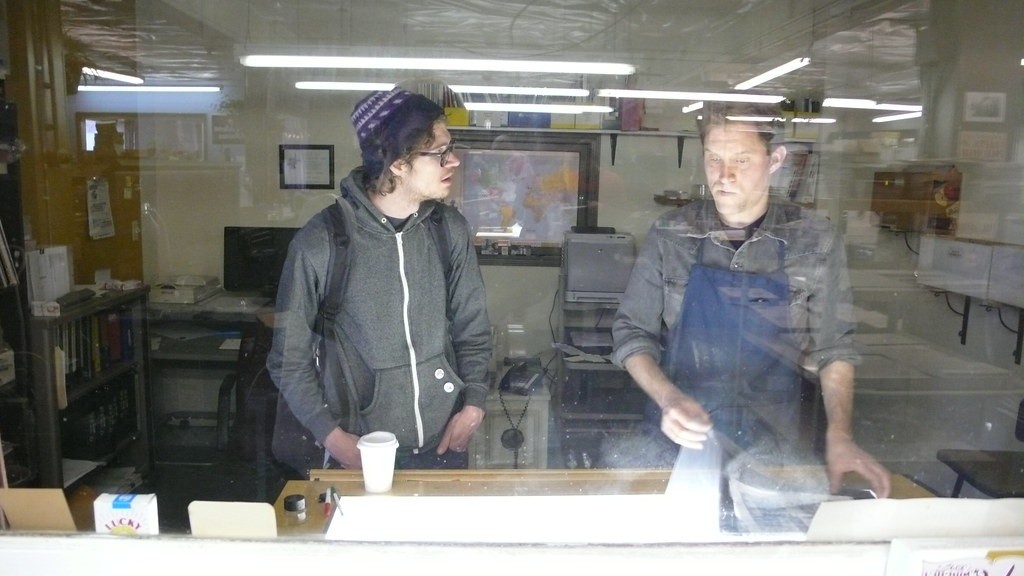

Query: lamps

[233, 45, 640, 75]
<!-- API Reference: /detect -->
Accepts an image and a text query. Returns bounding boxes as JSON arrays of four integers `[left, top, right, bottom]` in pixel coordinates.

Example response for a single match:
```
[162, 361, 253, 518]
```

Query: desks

[272, 457, 939, 543]
[744, 324, 1011, 446]
[146, 290, 272, 453]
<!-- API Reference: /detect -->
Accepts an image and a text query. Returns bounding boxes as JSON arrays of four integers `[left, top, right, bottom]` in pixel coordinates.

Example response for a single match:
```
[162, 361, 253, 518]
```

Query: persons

[266, 88, 493, 469]
[610, 88, 891, 500]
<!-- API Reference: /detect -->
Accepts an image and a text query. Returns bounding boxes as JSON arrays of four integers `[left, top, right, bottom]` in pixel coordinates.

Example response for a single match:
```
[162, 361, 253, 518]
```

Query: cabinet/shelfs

[27, 283, 157, 502]
[465, 383, 551, 470]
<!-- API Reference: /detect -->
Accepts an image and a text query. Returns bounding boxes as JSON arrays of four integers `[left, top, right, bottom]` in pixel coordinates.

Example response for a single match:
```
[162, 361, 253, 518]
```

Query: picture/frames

[279, 145, 334, 189]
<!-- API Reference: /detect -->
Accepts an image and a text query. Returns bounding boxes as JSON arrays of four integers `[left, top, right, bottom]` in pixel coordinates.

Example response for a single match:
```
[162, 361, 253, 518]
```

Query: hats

[350, 89, 445, 168]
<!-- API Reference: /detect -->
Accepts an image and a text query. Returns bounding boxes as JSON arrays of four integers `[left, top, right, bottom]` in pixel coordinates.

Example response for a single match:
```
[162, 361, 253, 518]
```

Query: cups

[356, 431, 400, 493]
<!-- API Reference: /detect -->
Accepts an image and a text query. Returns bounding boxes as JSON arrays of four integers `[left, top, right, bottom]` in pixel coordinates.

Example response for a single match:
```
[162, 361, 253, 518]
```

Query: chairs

[935, 403, 1024, 499]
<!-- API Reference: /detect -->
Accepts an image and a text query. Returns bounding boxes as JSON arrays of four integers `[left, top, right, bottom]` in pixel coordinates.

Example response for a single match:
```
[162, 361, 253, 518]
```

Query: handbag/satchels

[271, 359, 351, 481]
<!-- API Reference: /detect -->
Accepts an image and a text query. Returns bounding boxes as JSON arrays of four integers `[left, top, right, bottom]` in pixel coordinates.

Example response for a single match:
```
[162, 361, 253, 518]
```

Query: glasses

[404, 138, 456, 167]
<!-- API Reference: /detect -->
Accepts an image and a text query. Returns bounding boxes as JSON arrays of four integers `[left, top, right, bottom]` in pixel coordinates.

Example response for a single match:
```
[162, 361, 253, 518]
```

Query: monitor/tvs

[223, 226, 302, 297]
[562, 229, 636, 303]
[433, 140, 589, 257]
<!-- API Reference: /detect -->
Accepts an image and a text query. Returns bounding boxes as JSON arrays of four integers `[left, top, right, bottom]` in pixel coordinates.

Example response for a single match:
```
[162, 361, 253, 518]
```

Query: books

[0, 221, 21, 288]
[52, 304, 144, 496]
[772, 146, 818, 204]
[401, 81, 466, 108]
